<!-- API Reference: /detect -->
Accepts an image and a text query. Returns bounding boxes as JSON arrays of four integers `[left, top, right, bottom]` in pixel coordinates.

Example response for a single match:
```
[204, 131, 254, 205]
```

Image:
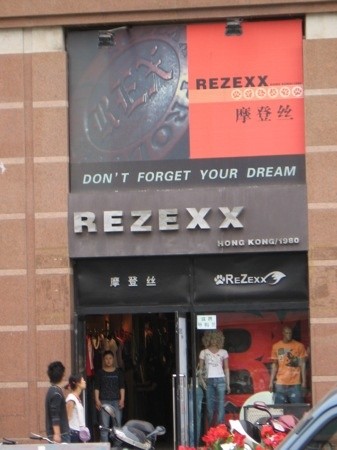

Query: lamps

[224, 18, 244, 35]
[97, 25, 130, 48]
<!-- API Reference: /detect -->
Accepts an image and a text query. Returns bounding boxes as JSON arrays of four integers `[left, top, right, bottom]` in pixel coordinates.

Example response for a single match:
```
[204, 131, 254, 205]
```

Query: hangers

[87, 330, 131, 338]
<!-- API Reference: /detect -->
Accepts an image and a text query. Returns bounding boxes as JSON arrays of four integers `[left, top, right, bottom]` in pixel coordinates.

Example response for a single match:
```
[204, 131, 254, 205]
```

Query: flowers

[179, 424, 287, 450]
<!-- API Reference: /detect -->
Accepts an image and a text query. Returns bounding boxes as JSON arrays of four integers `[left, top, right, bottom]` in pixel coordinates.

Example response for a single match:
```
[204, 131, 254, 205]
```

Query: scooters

[220, 401, 301, 450]
[98, 403, 167, 450]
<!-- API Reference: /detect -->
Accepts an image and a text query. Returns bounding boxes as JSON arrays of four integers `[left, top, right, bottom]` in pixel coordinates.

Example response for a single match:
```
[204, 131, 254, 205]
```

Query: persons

[268, 326, 308, 403]
[45, 361, 72, 444]
[93, 351, 126, 450]
[64, 372, 87, 443]
[198, 327, 230, 430]
